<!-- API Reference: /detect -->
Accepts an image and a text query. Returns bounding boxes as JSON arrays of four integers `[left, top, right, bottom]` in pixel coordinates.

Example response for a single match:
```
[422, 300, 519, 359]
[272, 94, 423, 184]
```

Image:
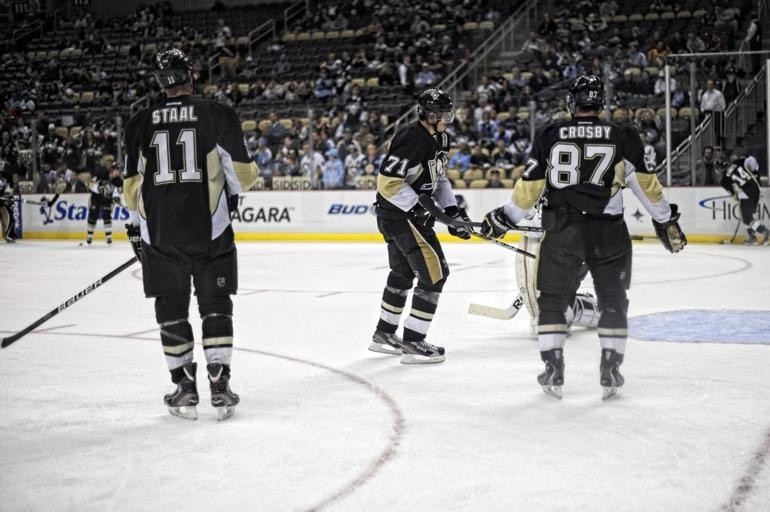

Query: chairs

[0, 0, 766, 185]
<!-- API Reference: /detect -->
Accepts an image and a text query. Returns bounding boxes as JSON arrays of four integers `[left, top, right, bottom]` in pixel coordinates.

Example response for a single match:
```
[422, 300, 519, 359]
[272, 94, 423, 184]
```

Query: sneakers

[373, 330, 403, 348]
[401, 341, 444, 356]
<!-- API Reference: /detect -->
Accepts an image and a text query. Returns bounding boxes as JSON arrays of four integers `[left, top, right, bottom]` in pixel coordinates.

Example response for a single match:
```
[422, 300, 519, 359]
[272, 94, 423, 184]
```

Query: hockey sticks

[0, 193, 59, 206]
[720, 219, 741, 244]
[418, 193, 681, 244]
[0, 256, 139, 348]
[468, 292, 525, 320]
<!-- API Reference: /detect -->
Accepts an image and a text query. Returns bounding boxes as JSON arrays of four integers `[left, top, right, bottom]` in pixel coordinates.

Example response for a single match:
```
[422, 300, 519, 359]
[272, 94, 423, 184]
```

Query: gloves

[481, 206, 517, 239]
[412, 201, 436, 227]
[652, 203, 687, 254]
[444, 205, 470, 240]
[124, 223, 142, 262]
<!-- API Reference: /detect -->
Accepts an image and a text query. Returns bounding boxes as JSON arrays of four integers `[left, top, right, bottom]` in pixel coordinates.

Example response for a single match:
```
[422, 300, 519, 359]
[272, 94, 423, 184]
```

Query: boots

[600, 350, 625, 386]
[206, 363, 240, 407]
[537, 348, 564, 385]
[761, 225, 770, 245]
[164, 362, 199, 407]
[745, 232, 758, 243]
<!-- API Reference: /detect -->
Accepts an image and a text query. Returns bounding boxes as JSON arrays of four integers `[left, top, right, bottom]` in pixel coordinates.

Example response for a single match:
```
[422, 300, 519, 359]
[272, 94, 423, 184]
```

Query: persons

[713, 156, 769, 246]
[370, 88, 473, 365]
[123, 48, 260, 420]
[516, 203, 599, 339]
[84, 154, 123, 246]
[0, 159, 17, 242]
[482, 74, 687, 401]
[0, 1, 769, 195]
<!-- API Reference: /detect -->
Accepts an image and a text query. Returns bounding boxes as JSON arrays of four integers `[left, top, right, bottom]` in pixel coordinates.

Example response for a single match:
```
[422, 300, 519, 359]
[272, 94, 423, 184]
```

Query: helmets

[566, 74, 604, 114]
[419, 88, 455, 123]
[714, 159, 729, 169]
[112, 161, 124, 171]
[152, 48, 193, 88]
